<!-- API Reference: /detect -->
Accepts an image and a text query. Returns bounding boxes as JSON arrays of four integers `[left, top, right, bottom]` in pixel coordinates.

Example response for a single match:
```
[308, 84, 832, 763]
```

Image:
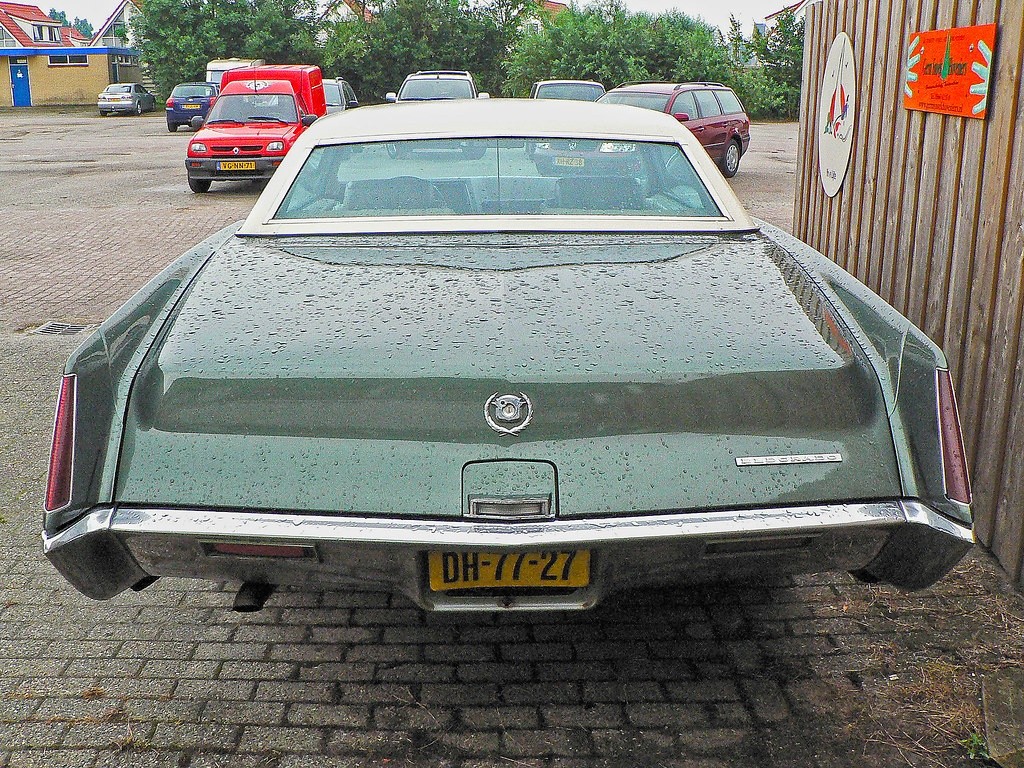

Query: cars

[95, 83, 157, 115]
[38, 97, 977, 626]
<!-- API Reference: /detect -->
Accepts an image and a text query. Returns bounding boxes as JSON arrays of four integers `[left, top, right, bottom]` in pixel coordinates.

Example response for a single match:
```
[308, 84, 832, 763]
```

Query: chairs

[342, 177, 446, 210]
[554, 174, 649, 212]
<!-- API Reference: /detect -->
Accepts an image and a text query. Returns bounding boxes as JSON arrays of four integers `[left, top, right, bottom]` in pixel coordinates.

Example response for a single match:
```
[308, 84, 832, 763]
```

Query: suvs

[164, 82, 219, 131]
[533, 80, 752, 181]
[320, 77, 359, 115]
[383, 70, 491, 161]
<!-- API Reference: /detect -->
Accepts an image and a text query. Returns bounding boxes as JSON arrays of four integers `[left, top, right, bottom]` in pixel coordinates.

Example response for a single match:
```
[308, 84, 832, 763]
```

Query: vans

[525, 81, 606, 163]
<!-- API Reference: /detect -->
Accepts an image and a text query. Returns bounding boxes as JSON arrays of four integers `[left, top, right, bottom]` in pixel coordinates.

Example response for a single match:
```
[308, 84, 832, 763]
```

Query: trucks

[207, 58, 265, 88]
[184, 65, 327, 193]
